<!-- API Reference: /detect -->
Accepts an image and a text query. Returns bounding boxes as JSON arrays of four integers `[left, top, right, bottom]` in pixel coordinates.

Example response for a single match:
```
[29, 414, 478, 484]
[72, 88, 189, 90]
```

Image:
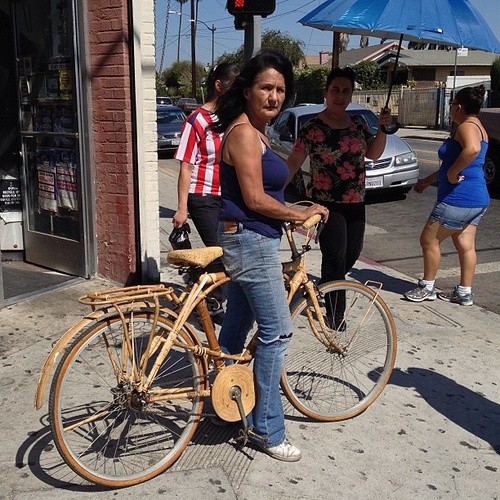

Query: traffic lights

[228, 0, 277, 14]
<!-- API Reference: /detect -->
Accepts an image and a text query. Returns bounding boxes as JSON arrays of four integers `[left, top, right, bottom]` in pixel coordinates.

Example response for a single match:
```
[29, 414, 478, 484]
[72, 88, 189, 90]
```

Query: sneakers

[404, 278, 437, 302]
[438, 285, 474, 306]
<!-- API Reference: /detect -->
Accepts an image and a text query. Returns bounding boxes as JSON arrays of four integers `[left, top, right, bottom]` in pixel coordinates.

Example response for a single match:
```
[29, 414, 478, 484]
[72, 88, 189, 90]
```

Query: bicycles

[36, 199, 399, 488]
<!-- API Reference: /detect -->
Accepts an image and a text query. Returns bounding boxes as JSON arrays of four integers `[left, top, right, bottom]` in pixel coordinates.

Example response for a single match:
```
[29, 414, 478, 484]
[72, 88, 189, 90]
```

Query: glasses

[449, 102, 458, 107]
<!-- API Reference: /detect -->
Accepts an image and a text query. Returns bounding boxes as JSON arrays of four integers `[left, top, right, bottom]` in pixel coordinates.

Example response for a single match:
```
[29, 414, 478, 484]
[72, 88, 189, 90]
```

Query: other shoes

[326, 313, 346, 331]
[197, 304, 226, 331]
[259, 440, 303, 462]
[211, 412, 232, 427]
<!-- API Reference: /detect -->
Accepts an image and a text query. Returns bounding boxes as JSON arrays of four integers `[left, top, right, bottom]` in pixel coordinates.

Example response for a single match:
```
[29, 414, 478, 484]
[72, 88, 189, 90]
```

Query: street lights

[188, 19, 217, 66]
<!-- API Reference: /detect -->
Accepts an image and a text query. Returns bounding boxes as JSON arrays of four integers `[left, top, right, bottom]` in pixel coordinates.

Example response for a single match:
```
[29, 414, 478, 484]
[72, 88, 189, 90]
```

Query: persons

[204, 50, 330, 462]
[172, 60, 242, 333]
[403, 85, 490, 305]
[286, 67, 393, 333]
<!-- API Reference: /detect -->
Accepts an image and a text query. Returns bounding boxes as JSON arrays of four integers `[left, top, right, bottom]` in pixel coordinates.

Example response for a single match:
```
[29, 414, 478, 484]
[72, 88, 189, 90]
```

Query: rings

[324, 207, 327, 211]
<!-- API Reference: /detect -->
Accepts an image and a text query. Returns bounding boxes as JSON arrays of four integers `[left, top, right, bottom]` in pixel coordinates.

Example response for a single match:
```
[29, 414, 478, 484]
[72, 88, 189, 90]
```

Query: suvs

[472, 107, 500, 198]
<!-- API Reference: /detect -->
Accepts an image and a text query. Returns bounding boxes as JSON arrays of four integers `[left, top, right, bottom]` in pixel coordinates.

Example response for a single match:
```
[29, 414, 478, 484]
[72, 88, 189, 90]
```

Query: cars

[263, 102, 420, 200]
[156, 96, 199, 151]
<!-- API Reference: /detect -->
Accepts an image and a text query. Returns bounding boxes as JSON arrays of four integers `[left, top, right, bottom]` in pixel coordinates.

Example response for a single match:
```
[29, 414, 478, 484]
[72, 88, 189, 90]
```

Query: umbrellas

[297, 0, 500, 135]
